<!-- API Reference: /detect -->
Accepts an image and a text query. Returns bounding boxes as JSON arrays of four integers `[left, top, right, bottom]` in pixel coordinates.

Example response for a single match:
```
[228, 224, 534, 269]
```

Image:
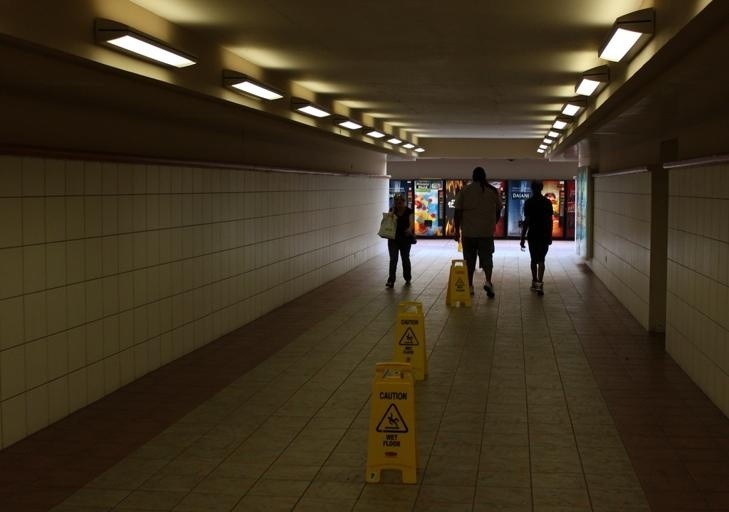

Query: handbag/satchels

[378, 208, 398, 239]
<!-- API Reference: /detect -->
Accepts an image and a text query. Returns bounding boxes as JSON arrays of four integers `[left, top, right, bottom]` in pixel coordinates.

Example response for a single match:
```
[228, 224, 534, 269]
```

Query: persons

[454, 167, 502, 298]
[384, 194, 414, 290]
[520, 179, 553, 296]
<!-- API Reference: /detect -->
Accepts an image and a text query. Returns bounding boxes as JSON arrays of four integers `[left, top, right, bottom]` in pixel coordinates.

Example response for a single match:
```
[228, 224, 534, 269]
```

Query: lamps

[534, 6, 659, 156]
[290, 101, 331, 117]
[97, 21, 198, 70]
[333, 116, 430, 158]
[224, 76, 283, 101]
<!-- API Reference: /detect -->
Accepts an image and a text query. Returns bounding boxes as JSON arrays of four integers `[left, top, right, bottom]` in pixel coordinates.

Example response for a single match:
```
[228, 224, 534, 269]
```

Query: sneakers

[470, 286, 474, 296]
[483, 280, 496, 298]
[386, 282, 394, 289]
[535, 282, 545, 295]
[530, 280, 538, 290]
[405, 281, 410, 288]
